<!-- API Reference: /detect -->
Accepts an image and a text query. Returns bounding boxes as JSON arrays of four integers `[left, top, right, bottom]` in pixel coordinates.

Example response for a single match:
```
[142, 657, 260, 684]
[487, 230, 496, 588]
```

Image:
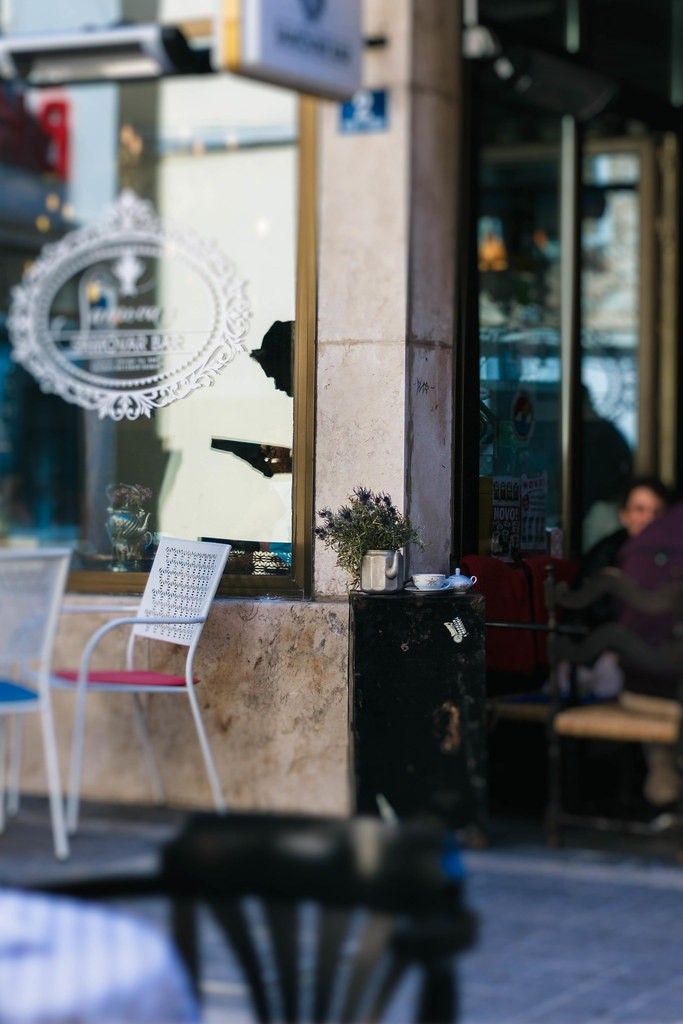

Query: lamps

[0, 22, 195, 88]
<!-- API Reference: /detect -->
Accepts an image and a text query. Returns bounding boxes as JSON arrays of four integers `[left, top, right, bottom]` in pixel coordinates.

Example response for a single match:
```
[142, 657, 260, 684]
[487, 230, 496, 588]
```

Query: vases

[105, 507, 152, 572]
[361, 550, 405, 594]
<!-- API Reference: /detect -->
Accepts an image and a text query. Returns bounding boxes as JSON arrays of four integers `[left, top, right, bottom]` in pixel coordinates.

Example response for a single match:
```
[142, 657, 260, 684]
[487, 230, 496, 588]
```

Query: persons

[573, 479, 683, 805]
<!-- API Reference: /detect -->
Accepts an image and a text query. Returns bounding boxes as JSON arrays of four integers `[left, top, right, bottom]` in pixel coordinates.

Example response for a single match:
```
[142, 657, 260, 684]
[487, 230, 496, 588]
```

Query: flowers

[106, 482, 154, 513]
[313, 486, 424, 595]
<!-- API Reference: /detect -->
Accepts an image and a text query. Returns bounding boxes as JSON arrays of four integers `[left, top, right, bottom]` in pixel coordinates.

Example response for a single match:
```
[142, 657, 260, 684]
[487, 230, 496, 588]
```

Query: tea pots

[445, 567, 477, 595]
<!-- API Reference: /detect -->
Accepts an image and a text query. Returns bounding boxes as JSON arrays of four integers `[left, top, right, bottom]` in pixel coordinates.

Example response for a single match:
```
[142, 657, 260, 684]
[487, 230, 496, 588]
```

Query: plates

[404, 585, 450, 595]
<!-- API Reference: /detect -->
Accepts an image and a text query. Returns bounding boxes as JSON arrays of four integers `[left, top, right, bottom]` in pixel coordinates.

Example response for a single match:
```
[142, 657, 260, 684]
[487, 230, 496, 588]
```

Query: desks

[0, 885, 200, 1024]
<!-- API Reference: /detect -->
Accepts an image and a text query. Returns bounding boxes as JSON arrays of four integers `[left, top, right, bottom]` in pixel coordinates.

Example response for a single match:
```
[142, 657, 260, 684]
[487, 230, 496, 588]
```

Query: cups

[412, 573, 451, 590]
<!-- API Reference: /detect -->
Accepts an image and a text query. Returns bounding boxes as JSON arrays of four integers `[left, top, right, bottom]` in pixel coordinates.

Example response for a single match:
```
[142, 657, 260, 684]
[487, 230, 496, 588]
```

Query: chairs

[7, 536, 233, 838]
[455, 554, 683, 855]
[0, 547, 76, 860]
[24, 806, 480, 1024]
